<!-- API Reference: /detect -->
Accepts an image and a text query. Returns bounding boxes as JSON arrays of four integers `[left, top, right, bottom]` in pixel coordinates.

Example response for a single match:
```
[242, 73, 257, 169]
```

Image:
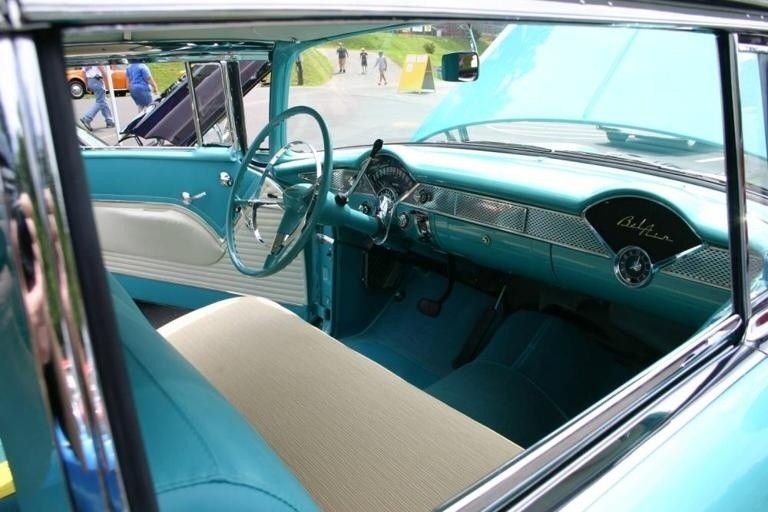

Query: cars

[0, 0, 767, 511]
[73, 57, 270, 152]
[67, 63, 131, 99]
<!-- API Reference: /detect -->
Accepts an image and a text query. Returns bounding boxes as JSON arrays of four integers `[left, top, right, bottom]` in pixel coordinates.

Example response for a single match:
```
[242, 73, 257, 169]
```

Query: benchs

[45, 267, 527, 511]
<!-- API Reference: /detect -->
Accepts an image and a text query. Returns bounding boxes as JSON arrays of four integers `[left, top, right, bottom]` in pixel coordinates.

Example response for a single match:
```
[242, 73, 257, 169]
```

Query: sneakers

[106, 123, 114, 128]
[80, 116, 92, 131]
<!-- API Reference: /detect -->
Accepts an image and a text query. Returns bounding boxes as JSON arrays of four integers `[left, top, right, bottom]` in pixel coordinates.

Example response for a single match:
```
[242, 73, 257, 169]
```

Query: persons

[294, 54, 304, 86]
[261, 73, 271, 86]
[372, 50, 387, 85]
[125, 63, 159, 114]
[359, 47, 369, 74]
[336, 42, 349, 73]
[79, 63, 115, 131]
